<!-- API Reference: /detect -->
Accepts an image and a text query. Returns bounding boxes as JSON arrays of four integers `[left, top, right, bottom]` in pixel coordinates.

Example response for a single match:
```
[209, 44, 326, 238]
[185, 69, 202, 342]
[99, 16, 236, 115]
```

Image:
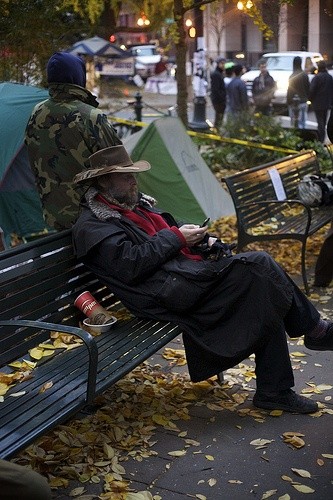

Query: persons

[25, 51, 124, 231]
[192, 68, 209, 98]
[91, 54, 103, 83]
[227, 64, 250, 116]
[71, 144, 333, 414]
[252, 60, 277, 122]
[312, 105, 333, 288]
[0, 459, 52, 500]
[211, 57, 227, 128]
[286, 57, 310, 129]
[310, 60, 333, 144]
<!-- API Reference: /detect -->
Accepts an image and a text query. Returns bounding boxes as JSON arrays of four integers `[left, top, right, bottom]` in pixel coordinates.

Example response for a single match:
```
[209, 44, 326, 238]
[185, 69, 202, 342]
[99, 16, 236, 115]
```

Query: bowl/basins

[83, 316, 117, 333]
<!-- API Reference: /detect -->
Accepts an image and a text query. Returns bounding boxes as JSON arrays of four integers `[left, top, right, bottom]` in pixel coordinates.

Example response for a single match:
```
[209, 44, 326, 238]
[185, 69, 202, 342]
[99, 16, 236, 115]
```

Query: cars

[127, 45, 163, 80]
[241, 51, 324, 112]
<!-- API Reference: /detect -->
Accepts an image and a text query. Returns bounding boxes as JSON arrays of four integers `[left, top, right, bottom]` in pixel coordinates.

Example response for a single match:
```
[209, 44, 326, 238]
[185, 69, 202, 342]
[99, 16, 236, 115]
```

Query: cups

[74, 291, 113, 325]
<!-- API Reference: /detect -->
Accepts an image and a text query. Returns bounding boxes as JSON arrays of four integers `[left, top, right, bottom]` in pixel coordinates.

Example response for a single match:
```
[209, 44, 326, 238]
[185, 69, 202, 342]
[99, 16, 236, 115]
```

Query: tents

[0, 81, 59, 251]
[119, 115, 236, 224]
[145, 70, 177, 95]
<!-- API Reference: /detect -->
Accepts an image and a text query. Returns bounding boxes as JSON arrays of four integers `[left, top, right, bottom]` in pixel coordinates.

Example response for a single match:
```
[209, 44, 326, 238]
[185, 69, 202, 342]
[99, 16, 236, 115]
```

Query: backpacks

[299, 173, 333, 208]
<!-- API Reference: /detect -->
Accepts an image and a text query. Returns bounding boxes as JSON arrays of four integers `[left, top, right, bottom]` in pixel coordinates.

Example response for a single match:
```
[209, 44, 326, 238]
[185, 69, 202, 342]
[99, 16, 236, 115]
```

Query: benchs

[0, 231, 237, 460]
[221, 149, 333, 297]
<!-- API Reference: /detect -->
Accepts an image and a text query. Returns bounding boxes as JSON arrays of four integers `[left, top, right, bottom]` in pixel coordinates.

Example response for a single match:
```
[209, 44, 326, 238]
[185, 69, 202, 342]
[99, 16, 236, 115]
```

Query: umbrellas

[62, 35, 135, 83]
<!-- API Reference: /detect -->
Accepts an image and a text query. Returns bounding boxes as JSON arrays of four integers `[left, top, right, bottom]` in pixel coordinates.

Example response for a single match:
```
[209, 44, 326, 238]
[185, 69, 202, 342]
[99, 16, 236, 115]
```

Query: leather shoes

[253, 389, 318, 414]
[304, 323, 333, 351]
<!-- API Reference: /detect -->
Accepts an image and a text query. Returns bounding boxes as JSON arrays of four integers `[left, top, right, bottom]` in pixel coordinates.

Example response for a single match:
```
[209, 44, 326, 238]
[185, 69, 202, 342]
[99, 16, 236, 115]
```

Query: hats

[73, 145, 151, 184]
